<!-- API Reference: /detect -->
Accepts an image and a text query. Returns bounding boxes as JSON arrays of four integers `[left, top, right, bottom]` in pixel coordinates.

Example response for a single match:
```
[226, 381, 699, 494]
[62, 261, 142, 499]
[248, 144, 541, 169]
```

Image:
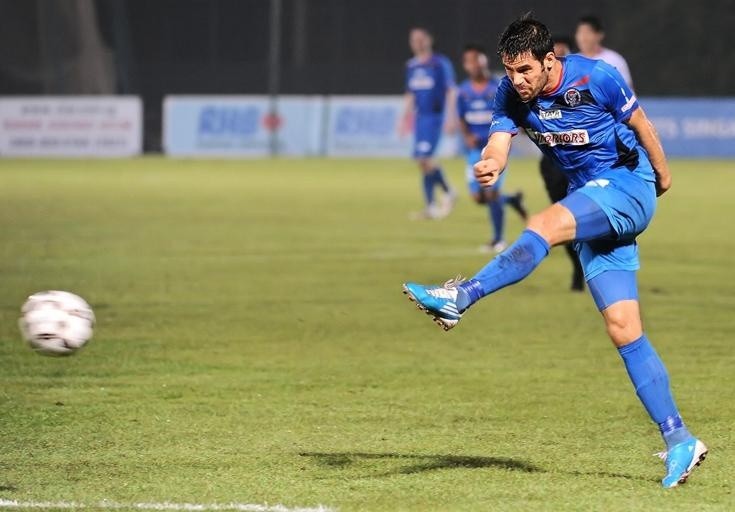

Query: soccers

[18, 291, 95, 357]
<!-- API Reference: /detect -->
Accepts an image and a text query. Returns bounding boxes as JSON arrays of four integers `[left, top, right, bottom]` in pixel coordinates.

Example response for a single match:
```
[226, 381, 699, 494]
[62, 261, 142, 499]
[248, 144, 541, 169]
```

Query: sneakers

[482, 240, 506, 252]
[410, 189, 456, 221]
[511, 193, 526, 219]
[402, 282, 469, 331]
[652, 438, 708, 488]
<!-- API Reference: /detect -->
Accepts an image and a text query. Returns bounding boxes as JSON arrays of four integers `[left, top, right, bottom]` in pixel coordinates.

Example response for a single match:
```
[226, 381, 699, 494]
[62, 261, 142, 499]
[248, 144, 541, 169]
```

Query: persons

[394, 23, 455, 221]
[541, 34, 584, 291]
[455, 45, 529, 255]
[573, 16, 635, 95]
[398, 10, 708, 491]
